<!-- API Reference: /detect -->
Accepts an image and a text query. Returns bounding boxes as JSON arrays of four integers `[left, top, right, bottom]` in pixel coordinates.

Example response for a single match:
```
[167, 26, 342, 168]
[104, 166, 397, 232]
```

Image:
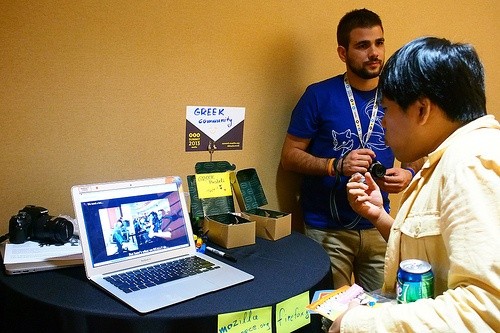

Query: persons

[279, 9, 416, 292]
[147, 212, 161, 248]
[328, 36, 500, 333]
[113, 219, 125, 254]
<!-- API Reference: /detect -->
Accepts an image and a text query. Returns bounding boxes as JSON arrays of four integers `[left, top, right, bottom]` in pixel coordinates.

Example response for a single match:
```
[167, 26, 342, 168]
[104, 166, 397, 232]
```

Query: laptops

[70, 176, 254, 313]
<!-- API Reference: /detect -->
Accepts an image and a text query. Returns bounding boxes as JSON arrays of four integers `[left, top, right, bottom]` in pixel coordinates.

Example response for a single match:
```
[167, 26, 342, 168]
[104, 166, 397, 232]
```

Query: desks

[0, 229, 334, 333]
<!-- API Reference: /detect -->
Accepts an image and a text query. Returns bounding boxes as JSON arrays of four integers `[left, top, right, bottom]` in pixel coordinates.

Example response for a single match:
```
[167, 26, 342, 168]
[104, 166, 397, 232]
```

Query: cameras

[9, 204, 74, 246]
[368, 160, 386, 178]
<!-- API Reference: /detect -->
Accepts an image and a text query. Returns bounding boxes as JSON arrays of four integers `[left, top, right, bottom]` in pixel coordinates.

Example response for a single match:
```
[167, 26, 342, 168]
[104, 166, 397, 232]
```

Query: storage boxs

[195, 161, 256, 249]
[230, 167, 292, 240]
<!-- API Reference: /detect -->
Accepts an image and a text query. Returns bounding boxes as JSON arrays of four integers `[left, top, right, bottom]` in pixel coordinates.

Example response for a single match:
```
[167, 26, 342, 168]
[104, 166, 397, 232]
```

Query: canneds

[396, 259, 435, 304]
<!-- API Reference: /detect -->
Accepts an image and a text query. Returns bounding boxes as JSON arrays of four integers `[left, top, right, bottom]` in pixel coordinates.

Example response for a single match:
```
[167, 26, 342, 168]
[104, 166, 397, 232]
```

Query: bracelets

[406, 168, 415, 177]
[328, 157, 346, 177]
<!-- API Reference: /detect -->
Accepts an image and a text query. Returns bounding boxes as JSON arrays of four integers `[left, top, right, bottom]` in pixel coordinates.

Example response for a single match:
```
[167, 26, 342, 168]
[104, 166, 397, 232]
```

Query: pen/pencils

[205, 246, 238, 262]
